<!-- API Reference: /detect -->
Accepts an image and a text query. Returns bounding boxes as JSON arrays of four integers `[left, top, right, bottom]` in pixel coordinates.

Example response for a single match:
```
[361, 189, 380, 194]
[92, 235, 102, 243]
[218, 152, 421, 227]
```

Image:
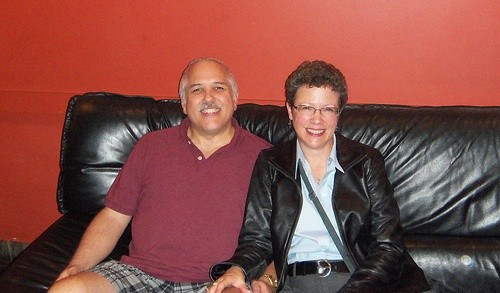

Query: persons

[47, 56, 278, 292]
[204, 58, 431, 293]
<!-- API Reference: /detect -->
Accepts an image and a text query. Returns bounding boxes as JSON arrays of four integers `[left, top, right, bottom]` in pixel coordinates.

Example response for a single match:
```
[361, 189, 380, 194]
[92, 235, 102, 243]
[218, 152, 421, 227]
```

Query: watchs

[260, 273, 278, 290]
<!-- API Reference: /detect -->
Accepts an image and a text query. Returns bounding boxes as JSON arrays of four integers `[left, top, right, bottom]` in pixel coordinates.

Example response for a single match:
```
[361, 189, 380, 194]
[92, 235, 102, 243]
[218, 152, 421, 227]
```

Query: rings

[212, 283, 217, 285]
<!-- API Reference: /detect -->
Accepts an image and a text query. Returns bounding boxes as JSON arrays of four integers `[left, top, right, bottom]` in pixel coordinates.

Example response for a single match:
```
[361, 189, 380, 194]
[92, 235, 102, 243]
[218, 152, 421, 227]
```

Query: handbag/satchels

[370, 249, 428, 292]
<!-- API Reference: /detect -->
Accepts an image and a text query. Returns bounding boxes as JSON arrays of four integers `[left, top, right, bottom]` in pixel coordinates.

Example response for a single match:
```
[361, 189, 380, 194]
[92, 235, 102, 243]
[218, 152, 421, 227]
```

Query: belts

[286, 260, 350, 276]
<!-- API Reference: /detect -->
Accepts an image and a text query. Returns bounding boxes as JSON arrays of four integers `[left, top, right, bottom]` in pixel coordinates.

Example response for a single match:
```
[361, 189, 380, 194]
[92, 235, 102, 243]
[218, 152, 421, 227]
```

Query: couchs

[0, 90, 497, 293]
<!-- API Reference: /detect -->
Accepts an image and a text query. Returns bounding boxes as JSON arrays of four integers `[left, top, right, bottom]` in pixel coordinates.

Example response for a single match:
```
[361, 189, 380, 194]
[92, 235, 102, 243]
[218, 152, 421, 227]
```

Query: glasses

[292, 103, 341, 118]
